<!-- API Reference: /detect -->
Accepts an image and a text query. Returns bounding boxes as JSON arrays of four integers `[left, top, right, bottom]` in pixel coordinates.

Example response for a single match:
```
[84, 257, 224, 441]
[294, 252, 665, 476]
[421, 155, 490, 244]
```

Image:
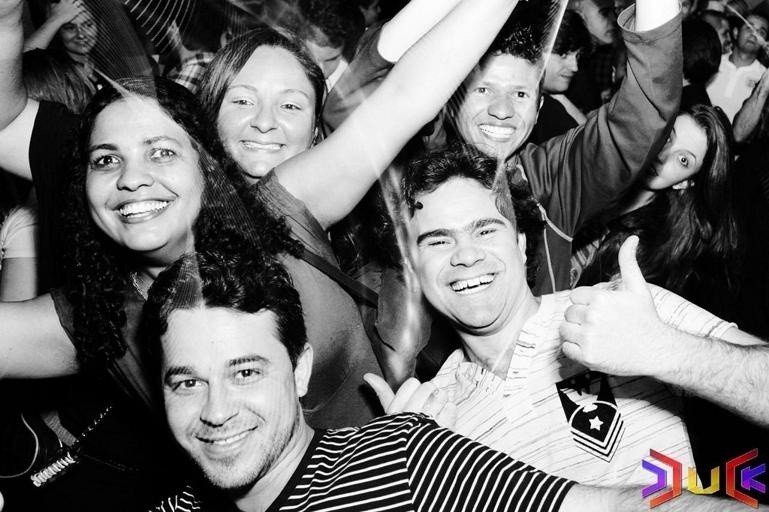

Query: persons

[0, 0, 769, 512]
